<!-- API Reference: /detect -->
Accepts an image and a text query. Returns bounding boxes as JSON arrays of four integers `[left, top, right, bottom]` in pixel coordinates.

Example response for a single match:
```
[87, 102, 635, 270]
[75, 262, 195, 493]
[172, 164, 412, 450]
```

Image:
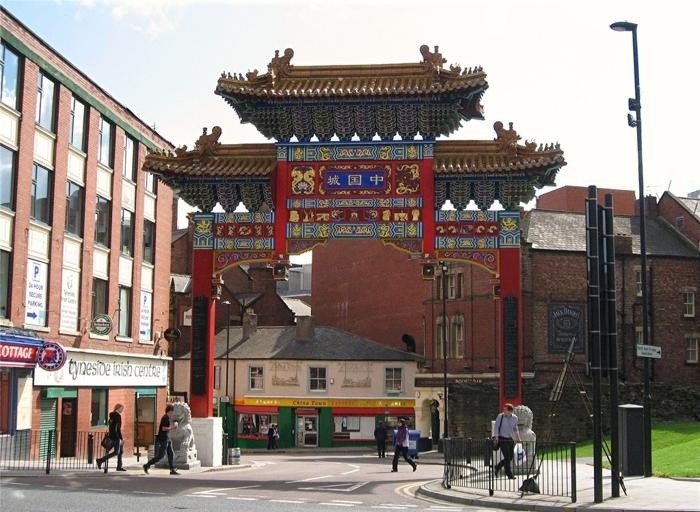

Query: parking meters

[86, 433, 94, 465]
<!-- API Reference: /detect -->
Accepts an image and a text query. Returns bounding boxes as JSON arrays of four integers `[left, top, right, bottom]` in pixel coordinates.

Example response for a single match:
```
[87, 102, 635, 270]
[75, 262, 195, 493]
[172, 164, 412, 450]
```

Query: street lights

[609, 21, 653, 476]
[220, 300, 230, 430]
[440, 262, 451, 436]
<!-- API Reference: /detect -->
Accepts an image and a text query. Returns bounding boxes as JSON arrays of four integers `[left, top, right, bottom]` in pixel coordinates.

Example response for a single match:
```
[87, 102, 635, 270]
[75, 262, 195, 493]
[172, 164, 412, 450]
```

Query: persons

[493, 402, 522, 479]
[375, 422, 388, 458]
[273, 425, 280, 448]
[143, 404, 181, 475]
[267, 424, 275, 449]
[96, 403, 127, 471]
[390, 417, 417, 472]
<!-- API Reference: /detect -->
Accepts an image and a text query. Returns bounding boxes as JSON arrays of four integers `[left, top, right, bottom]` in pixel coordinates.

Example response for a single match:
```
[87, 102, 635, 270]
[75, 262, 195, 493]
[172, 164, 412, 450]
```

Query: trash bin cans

[392, 430, 420, 459]
[615, 405, 643, 477]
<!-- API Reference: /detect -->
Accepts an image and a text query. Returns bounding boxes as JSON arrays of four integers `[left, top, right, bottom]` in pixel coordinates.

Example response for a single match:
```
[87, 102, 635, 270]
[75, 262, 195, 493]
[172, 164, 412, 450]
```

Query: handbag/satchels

[101, 434, 115, 450]
[489, 436, 500, 450]
[514, 442, 526, 466]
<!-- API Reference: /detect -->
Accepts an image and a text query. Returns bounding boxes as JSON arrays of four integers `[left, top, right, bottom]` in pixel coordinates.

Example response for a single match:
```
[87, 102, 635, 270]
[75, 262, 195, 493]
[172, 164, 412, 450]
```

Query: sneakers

[391, 469, 397, 472]
[413, 464, 416, 471]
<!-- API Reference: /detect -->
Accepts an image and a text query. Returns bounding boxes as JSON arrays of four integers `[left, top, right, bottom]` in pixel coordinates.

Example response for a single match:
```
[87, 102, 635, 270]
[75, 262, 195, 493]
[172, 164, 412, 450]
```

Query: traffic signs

[635, 343, 662, 360]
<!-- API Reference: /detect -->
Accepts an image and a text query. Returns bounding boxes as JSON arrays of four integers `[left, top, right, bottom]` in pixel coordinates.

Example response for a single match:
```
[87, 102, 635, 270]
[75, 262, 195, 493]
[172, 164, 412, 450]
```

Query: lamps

[437, 392, 444, 400]
[330, 377, 334, 385]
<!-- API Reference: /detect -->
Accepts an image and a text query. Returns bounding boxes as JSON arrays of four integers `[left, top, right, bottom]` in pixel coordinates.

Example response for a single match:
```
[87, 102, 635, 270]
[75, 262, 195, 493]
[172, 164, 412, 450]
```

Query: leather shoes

[495, 465, 499, 478]
[170, 471, 179, 475]
[144, 464, 148, 472]
[117, 467, 126, 470]
[97, 458, 102, 469]
[508, 475, 516, 479]
